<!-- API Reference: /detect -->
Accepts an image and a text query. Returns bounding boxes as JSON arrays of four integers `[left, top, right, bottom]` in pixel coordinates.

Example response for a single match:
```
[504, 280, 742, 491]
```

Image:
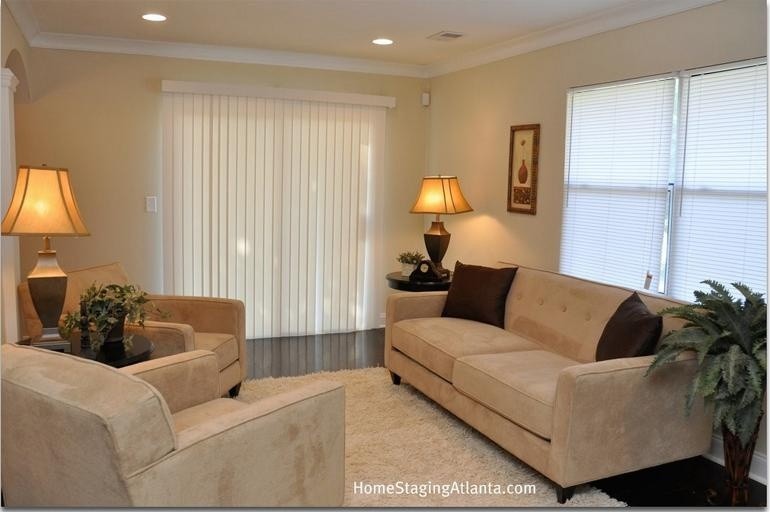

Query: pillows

[596, 291, 663, 362]
[441, 260, 518, 329]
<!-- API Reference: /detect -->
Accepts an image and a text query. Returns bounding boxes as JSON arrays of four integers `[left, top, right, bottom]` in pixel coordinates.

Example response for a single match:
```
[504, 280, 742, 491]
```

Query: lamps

[0, 164, 91, 341]
[409, 175, 473, 280]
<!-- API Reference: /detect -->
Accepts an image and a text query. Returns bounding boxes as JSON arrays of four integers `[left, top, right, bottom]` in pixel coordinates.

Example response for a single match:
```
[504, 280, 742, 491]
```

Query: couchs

[384, 261, 713, 503]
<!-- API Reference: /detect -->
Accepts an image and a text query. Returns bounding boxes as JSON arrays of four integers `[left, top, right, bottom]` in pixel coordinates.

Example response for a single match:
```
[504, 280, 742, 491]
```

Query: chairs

[18, 262, 246, 398]
[0, 343, 345, 508]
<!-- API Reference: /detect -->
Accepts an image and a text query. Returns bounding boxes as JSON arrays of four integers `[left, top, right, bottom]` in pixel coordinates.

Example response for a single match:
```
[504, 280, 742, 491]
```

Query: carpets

[225, 367, 628, 507]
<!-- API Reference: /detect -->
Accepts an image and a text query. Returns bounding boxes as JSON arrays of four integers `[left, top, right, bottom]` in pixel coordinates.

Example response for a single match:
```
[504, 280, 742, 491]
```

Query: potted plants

[396, 252, 425, 277]
[60, 280, 172, 352]
[642, 277, 767, 508]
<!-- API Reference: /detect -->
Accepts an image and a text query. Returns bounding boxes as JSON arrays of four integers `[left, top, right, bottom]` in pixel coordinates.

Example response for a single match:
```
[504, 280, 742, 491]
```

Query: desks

[385, 271, 452, 292]
[17, 332, 155, 368]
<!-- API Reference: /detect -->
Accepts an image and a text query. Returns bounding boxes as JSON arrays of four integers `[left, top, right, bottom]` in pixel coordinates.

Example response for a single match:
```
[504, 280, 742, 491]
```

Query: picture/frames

[507, 124, 540, 215]
